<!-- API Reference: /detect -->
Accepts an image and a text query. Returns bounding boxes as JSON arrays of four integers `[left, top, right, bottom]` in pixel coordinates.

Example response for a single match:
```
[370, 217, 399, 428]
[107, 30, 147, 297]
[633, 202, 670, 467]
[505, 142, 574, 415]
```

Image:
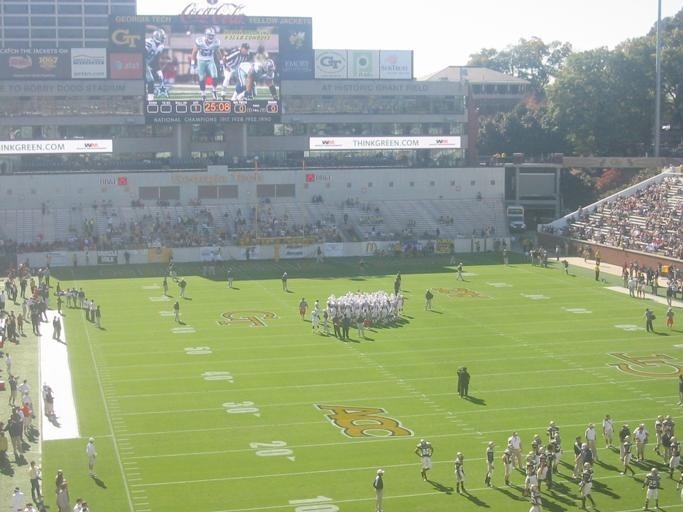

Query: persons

[308, 288, 405, 343]
[410, 412, 682, 511]
[281, 271, 288, 290]
[217, 41, 251, 99]
[455, 262, 464, 281]
[675, 373, 683, 407]
[424, 289, 433, 312]
[522, 162, 683, 302]
[171, 301, 179, 321]
[0, 264, 101, 511]
[252, 45, 267, 62]
[666, 307, 674, 328]
[189, 28, 222, 100]
[1, 147, 508, 174]
[144, 29, 166, 101]
[298, 296, 307, 320]
[230, 60, 278, 101]
[177, 277, 187, 297]
[456, 366, 463, 392]
[396, 270, 401, 279]
[459, 366, 470, 398]
[393, 277, 399, 296]
[372, 467, 386, 512]
[642, 308, 654, 332]
[161, 277, 168, 294]
[167, 264, 176, 281]
[226, 270, 233, 288]
[2, 195, 525, 269]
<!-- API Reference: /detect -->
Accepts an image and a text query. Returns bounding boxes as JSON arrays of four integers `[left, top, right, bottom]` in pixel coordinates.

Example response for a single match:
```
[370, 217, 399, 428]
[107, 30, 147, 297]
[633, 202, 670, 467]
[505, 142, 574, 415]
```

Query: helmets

[508, 414, 677, 493]
[456, 452, 463, 459]
[488, 441, 495, 449]
[420, 439, 427, 446]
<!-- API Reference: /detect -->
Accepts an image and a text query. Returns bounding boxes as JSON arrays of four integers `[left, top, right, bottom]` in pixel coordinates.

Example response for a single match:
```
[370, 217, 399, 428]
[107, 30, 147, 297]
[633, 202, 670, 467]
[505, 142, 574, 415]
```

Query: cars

[509, 221, 527, 233]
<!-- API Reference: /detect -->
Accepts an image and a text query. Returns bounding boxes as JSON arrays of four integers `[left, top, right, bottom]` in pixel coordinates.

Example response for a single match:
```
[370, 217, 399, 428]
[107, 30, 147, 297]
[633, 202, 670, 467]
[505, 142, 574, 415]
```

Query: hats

[375, 468, 385, 475]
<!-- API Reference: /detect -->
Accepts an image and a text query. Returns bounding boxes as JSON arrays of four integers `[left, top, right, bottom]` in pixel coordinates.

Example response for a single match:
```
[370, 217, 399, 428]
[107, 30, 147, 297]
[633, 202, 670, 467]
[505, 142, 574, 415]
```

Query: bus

[506, 206, 524, 223]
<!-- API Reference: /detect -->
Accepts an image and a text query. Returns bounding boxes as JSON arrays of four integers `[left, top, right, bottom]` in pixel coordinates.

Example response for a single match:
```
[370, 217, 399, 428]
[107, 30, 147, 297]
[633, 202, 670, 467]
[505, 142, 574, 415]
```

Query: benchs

[569, 175, 683, 259]
